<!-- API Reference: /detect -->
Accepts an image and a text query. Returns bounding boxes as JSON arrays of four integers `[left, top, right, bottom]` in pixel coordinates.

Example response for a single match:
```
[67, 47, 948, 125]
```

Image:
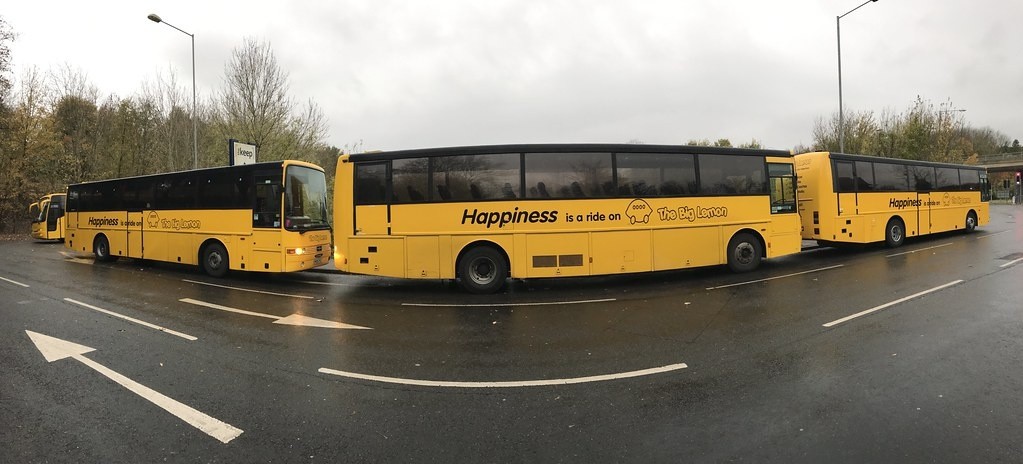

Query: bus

[332, 144, 805, 295]
[775, 149, 990, 249]
[64, 159, 332, 278]
[29, 193, 67, 243]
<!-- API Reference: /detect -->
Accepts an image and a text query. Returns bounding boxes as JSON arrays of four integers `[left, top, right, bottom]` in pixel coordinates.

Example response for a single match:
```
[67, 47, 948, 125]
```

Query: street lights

[147, 13, 198, 170]
[836, 0, 881, 155]
[936, 109, 967, 163]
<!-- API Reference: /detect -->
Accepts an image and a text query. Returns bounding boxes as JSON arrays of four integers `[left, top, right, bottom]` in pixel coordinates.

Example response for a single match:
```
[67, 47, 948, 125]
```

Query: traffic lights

[1016, 172, 1020, 185]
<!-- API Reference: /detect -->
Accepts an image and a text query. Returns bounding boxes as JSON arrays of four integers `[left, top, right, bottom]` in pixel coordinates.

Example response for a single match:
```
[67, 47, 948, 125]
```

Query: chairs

[360, 178, 758, 204]
